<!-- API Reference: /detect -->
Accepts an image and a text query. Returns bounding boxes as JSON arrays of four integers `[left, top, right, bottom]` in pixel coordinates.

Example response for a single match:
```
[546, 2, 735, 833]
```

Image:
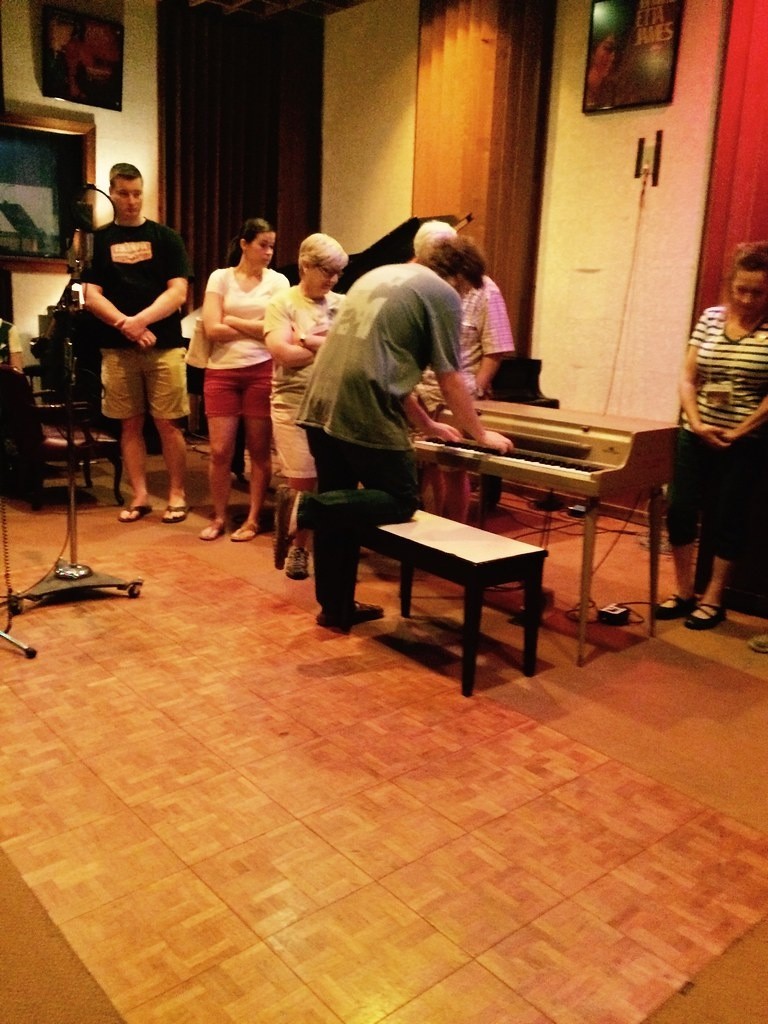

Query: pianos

[277, 213, 476, 295]
[415, 398, 684, 668]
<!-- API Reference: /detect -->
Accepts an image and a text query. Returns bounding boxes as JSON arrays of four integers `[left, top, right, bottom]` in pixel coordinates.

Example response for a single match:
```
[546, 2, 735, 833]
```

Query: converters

[598, 604, 630, 625]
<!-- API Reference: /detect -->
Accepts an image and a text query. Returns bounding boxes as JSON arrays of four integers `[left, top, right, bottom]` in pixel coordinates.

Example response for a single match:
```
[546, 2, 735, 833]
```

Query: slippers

[162, 504, 190, 522]
[199, 521, 226, 540]
[117, 505, 151, 522]
[231, 522, 258, 541]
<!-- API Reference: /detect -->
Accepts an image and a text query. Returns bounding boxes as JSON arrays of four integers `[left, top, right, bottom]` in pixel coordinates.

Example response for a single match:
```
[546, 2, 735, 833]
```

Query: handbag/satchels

[184, 317, 212, 369]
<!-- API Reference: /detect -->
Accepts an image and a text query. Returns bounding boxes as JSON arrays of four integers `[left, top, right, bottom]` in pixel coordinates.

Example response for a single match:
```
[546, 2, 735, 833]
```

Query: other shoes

[748, 635, 768, 653]
[685, 604, 725, 630]
[274, 484, 296, 570]
[655, 593, 698, 620]
[317, 600, 383, 626]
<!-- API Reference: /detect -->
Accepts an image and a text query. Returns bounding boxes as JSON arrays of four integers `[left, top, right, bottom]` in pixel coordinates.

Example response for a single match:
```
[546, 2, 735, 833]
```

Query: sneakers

[286, 546, 309, 580]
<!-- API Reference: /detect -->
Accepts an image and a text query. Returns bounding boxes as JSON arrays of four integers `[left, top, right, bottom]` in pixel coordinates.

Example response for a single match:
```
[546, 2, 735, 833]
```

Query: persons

[201, 217, 290, 542]
[412, 219, 516, 524]
[263, 233, 350, 579]
[0, 320, 25, 375]
[655, 242, 768, 630]
[272, 237, 511, 630]
[81, 163, 192, 524]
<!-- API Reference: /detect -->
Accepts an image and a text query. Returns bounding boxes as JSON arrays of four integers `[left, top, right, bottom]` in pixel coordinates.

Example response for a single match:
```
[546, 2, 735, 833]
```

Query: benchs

[359, 509, 550, 698]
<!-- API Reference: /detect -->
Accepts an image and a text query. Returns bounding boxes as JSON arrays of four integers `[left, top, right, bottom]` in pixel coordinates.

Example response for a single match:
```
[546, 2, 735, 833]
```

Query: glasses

[315, 263, 343, 279]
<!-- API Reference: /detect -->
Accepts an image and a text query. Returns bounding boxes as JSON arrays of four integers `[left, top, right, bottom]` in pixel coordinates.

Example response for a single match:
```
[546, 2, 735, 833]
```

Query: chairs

[0, 363, 126, 512]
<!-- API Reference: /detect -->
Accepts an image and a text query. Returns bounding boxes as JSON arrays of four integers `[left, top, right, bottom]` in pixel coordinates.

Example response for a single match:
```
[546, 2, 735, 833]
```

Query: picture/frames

[582, 0, 684, 114]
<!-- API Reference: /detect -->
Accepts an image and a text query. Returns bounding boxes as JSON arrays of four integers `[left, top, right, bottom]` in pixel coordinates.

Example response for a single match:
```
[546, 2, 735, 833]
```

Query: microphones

[73, 228, 86, 268]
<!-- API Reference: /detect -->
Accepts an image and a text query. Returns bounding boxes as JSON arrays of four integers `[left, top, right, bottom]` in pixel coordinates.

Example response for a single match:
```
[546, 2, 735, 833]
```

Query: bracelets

[299, 333, 306, 349]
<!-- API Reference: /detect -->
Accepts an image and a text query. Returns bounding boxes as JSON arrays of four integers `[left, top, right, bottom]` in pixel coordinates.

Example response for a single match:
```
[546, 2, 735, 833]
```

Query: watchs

[474, 387, 491, 401]
